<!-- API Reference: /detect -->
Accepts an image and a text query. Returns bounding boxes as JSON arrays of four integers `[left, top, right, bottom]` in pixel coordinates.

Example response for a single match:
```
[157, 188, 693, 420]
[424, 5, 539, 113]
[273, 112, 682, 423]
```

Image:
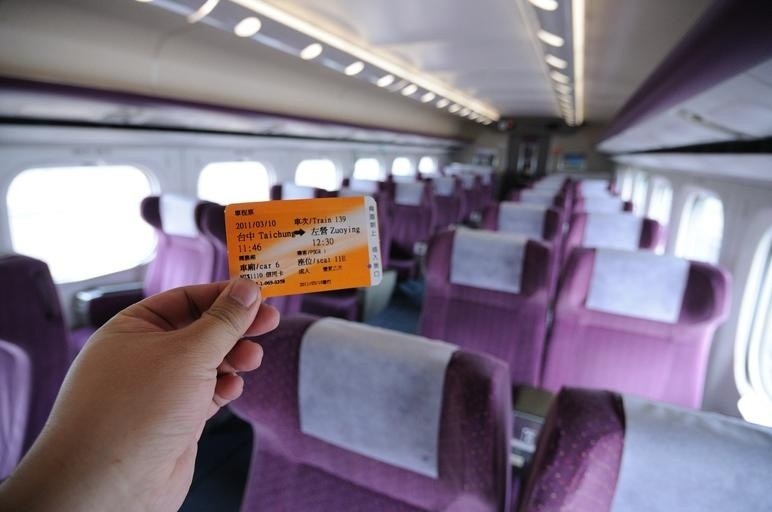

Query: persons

[0, 276, 281, 512]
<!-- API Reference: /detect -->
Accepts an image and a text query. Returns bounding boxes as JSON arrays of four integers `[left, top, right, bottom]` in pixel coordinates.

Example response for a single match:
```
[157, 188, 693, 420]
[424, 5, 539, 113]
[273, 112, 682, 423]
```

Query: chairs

[485, 172, 665, 253]
[0, 250, 68, 483]
[540, 250, 730, 410]
[65, 193, 228, 366]
[419, 226, 558, 389]
[515, 387, 772, 512]
[226, 316, 514, 512]
[262, 149, 492, 319]
